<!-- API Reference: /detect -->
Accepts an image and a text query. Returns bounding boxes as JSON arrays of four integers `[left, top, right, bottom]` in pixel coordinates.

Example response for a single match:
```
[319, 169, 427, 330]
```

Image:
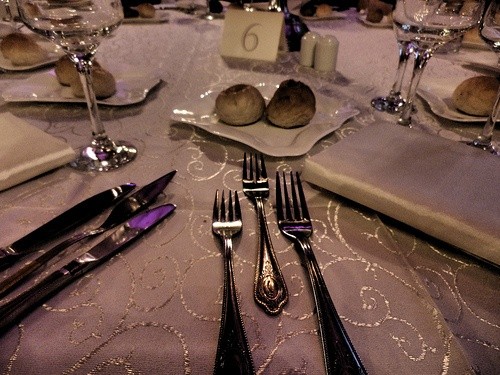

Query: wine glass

[369, 0, 500, 159]
[8, 0, 139, 173]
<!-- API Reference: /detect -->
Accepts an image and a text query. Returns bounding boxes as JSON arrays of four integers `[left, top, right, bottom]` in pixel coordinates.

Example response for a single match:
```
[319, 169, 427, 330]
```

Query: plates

[288, 8, 347, 19]
[416, 79, 500, 123]
[169, 77, 361, 157]
[463, 51, 500, 72]
[0, 20, 161, 107]
[357, 15, 392, 27]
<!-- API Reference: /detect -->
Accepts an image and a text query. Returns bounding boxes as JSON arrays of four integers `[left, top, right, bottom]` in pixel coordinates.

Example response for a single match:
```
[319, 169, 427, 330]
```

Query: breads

[55, 51, 117, 99]
[137, 3, 154, 18]
[265, 79, 316, 129]
[215, 83, 265, 126]
[366, 7, 384, 24]
[300, 2, 332, 17]
[0, 32, 46, 66]
[452, 76, 500, 117]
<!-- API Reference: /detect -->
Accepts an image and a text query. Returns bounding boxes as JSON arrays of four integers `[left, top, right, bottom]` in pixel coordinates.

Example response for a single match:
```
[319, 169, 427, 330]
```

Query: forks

[211, 189, 257, 375]
[241, 151, 290, 315]
[276, 171, 369, 375]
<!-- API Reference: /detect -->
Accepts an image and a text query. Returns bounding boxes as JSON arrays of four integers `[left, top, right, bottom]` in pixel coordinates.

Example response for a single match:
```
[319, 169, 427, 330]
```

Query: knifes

[0, 169, 178, 300]
[0, 203, 177, 339]
[0, 182, 136, 279]
